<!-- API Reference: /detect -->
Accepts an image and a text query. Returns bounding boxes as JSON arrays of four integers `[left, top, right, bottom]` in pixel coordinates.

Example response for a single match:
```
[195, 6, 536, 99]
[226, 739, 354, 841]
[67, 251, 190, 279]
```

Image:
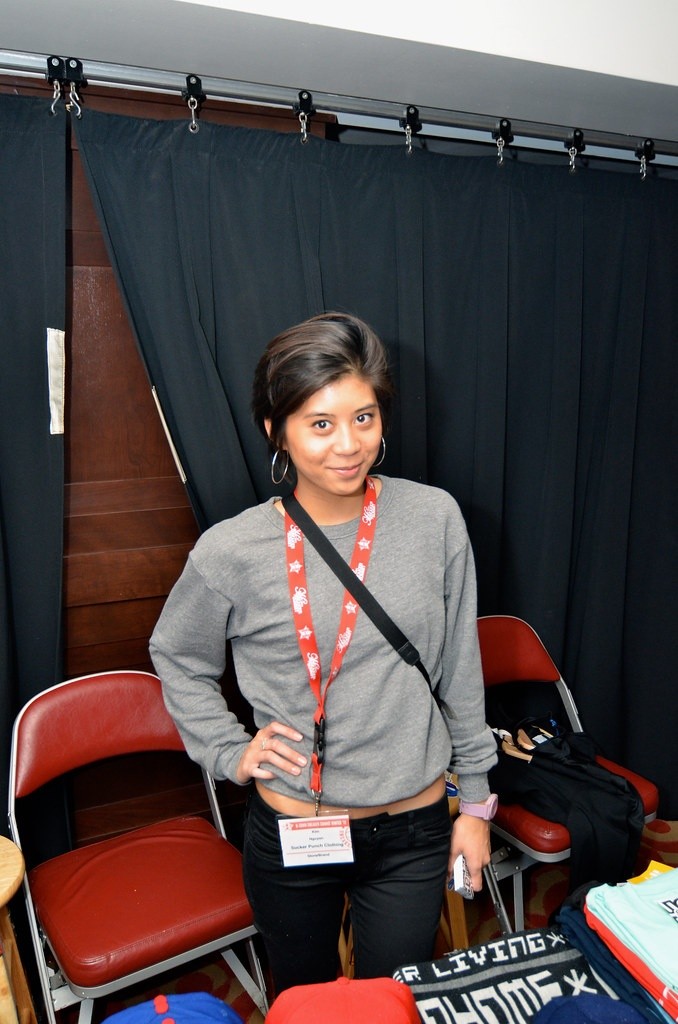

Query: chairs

[473, 614, 661, 936]
[7, 671, 270, 1024]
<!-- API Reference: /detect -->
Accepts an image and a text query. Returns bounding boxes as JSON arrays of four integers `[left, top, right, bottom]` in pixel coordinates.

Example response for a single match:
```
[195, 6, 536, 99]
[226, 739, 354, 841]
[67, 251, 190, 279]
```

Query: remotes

[453, 853, 474, 899]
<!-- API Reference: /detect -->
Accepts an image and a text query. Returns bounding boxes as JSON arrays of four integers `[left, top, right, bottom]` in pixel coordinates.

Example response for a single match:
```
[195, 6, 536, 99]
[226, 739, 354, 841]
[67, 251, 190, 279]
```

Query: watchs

[459, 793, 498, 820]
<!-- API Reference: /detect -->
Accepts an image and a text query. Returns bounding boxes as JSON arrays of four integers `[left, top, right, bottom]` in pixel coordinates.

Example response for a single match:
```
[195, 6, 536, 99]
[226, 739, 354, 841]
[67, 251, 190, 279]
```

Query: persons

[149, 313, 498, 997]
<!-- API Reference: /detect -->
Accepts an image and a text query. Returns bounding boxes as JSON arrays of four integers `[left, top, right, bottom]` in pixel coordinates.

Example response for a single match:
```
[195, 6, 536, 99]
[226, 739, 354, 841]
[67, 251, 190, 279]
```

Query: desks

[0, 835, 37, 1024]
[336, 773, 469, 979]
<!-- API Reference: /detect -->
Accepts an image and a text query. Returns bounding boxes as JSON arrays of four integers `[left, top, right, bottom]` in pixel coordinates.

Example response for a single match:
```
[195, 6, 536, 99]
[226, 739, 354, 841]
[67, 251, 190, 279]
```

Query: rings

[262, 738, 267, 751]
